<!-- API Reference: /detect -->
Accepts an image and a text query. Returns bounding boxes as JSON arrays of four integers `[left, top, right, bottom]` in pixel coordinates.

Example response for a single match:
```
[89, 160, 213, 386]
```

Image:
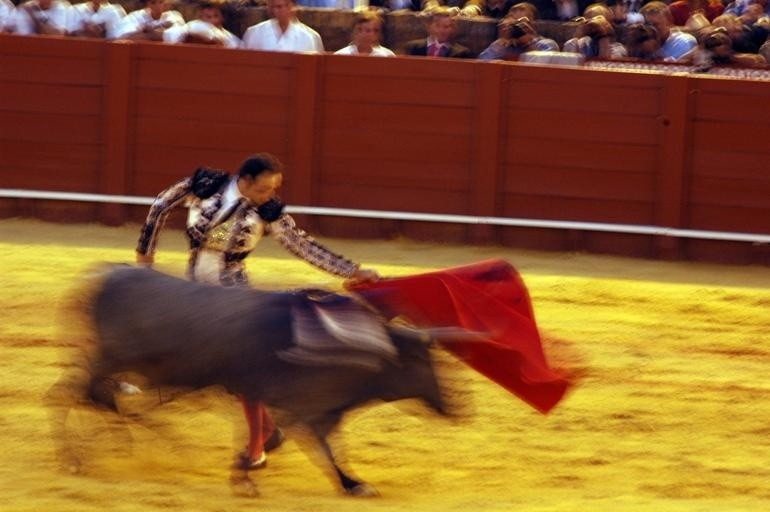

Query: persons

[479, 3, 560, 61]
[400, 6, 471, 60]
[242, 3, 326, 53]
[0, 0, 244, 49]
[563, 1, 770, 70]
[136, 153, 380, 473]
[333, 13, 395, 58]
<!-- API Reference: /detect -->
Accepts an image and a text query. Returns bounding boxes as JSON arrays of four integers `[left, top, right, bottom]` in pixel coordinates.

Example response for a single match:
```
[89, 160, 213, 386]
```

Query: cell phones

[506, 19, 526, 39]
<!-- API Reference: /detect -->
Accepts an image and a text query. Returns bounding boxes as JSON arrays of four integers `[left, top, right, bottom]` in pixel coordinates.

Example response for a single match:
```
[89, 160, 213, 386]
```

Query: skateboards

[44, 259, 475, 499]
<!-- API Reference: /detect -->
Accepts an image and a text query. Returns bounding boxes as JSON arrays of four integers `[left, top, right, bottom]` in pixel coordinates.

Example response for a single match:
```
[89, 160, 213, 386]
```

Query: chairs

[237, 453, 265, 471]
[263, 429, 287, 454]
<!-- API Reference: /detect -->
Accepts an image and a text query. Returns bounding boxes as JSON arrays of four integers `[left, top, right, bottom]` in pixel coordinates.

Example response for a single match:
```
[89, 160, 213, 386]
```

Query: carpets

[428, 44, 438, 56]
